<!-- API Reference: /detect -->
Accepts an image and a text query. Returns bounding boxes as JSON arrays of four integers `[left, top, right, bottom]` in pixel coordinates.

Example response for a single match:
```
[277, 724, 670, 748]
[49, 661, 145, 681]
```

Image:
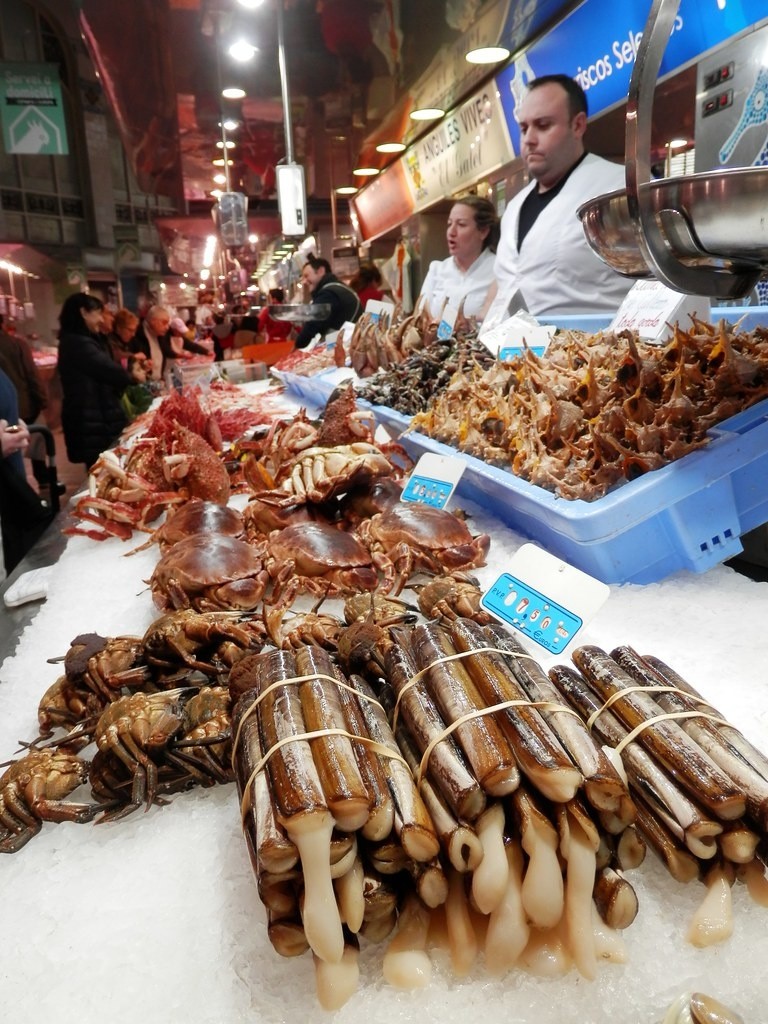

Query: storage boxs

[269, 319, 496, 433]
[241, 340, 295, 366]
[404, 305, 767, 585]
[171, 356, 268, 397]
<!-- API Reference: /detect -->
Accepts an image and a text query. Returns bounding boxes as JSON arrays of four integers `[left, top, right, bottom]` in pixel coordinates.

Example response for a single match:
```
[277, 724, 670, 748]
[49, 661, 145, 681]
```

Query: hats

[171, 318, 189, 333]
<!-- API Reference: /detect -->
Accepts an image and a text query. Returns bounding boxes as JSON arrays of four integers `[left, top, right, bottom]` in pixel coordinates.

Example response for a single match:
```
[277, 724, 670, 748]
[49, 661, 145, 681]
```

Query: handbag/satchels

[23, 411, 47, 460]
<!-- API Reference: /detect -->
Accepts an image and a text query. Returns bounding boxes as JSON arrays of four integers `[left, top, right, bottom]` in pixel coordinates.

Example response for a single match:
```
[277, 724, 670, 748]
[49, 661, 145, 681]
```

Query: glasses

[125, 327, 137, 333]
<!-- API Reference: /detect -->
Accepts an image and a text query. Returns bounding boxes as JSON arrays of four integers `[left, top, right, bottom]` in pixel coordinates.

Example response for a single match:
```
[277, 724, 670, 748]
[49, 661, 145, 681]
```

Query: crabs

[0, 299, 768, 854]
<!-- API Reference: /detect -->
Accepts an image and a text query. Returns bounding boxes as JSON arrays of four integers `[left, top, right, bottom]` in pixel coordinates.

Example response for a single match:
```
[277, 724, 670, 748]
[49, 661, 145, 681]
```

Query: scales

[218, 193, 260, 317]
[0, 294, 42, 340]
[577, 0, 768, 299]
[268, 165, 333, 324]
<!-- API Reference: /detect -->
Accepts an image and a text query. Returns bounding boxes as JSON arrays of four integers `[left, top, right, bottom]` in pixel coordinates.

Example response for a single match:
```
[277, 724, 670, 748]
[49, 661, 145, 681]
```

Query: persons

[413, 195, 500, 330]
[0, 289, 297, 577]
[349, 263, 388, 312]
[294, 258, 364, 349]
[493, 73, 657, 316]
